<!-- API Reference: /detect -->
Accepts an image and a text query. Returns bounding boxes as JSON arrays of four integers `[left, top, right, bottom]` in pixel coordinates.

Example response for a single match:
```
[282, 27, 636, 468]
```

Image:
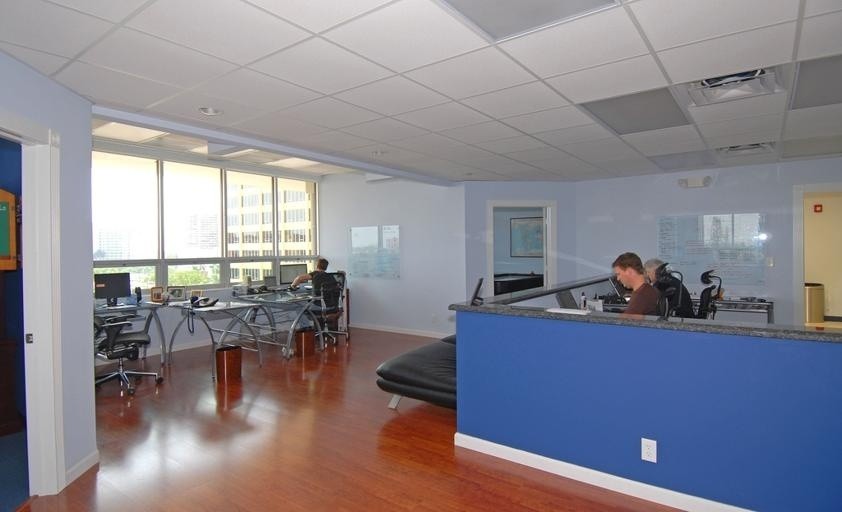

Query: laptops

[609, 275, 630, 296]
[556, 290, 579, 309]
[263, 276, 285, 291]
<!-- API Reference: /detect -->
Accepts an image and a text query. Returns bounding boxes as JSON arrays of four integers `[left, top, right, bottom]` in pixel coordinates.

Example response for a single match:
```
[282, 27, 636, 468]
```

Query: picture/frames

[167, 286, 185, 301]
[510, 216, 544, 257]
[0, 189, 17, 270]
[150, 287, 163, 302]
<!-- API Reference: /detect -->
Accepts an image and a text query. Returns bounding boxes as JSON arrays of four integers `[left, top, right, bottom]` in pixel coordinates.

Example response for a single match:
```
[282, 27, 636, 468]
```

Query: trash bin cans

[216, 346, 241, 383]
[296, 328, 315, 357]
[804, 283, 824, 323]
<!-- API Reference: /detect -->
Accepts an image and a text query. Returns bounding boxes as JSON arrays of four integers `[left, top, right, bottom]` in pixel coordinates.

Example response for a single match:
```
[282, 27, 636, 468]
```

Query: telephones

[192, 297, 219, 307]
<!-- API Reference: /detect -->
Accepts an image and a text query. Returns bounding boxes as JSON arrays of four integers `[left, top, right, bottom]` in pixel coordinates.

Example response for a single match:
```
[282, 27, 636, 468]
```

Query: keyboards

[107, 304, 137, 309]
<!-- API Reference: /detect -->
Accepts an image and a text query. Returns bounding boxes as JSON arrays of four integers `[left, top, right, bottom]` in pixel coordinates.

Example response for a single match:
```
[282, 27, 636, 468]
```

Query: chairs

[376, 331, 456, 410]
[651, 263, 721, 319]
[305, 273, 349, 344]
[94, 314, 163, 394]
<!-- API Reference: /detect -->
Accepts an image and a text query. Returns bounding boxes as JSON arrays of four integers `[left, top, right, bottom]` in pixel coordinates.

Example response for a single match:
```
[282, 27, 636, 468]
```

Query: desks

[94, 302, 167, 364]
[141, 301, 262, 380]
[217, 295, 325, 358]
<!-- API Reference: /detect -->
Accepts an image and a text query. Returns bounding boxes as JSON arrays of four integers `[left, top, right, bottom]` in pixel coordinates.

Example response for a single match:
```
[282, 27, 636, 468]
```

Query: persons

[290, 258, 340, 328]
[643, 257, 696, 317]
[611, 251, 658, 316]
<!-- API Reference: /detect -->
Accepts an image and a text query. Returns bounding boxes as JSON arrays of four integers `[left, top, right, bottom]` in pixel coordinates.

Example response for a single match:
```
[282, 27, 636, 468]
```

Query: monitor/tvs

[94, 273, 130, 308]
[280, 264, 307, 284]
